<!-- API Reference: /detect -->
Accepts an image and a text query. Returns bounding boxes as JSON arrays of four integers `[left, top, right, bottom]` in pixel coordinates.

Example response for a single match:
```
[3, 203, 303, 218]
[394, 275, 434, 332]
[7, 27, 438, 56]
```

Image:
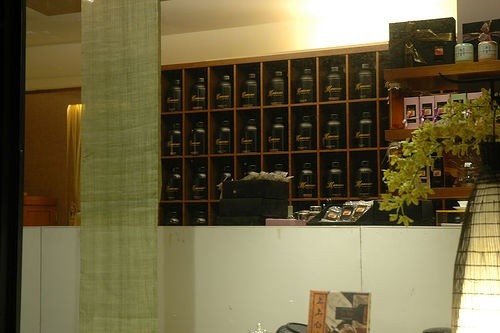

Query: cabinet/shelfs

[157, 43, 495, 224]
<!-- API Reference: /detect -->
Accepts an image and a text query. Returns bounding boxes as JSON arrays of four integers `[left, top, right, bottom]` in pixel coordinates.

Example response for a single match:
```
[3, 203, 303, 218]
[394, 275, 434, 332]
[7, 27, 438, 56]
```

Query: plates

[440, 222, 463, 227]
[453, 207, 467, 209]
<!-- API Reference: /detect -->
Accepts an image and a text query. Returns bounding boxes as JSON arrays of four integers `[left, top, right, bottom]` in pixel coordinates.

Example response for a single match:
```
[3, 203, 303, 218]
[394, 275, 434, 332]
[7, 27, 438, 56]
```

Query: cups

[457, 201, 469, 207]
[309, 206, 322, 216]
[293, 211, 309, 225]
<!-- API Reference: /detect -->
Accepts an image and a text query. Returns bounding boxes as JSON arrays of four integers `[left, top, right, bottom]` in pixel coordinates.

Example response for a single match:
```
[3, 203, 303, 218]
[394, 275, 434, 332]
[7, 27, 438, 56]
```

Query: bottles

[296, 117, 312, 151]
[355, 112, 370, 146]
[354, 159, 374, 197]
[464, 175, 472, 187]
[267, 71, 285, 104]
[196, 211, 206, 225]
[167, 80, 181, 112]
[190, 77, 206, 110]
[325, 161, 344, 199]
[240, 73, 259, 107]
[168, 211, 179, 223]
[268, 117, 284, 151]
[216, 76, 232, 109]
[324, 66, 342, 100]
[216, 119, 230, 153]
[166, 166, 181, 199]
[192, 167, 207, 200]
[297, 69, 313, 102]
[215, 161, 283, 199]
[357, 64, 372, 98]
[191, 116, 205, 154]
[297, 164, 314, 199]
[241, 119, 258, 151]
[323, 112, 340, 149]
[167, 123, 181, 155]
